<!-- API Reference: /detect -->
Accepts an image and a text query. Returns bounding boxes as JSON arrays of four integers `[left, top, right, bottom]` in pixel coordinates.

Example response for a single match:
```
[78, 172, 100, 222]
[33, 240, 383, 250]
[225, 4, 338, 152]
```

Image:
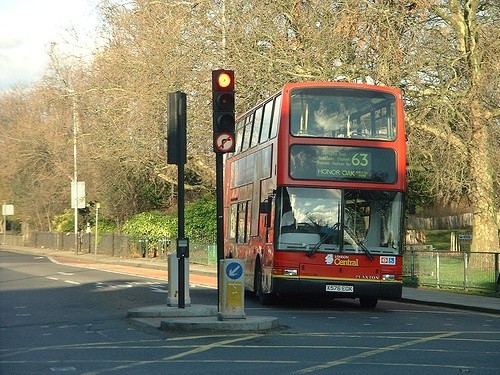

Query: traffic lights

[211, 69, 236, 132]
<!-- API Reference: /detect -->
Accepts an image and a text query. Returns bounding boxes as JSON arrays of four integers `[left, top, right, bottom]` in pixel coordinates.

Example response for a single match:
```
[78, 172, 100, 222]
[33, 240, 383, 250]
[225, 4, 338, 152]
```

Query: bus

[224, 81, 408, 310]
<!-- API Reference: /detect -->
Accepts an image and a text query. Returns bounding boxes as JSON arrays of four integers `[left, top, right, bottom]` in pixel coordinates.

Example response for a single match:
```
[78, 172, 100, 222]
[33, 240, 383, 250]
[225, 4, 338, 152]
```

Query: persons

[282, 196, 315, 228]
